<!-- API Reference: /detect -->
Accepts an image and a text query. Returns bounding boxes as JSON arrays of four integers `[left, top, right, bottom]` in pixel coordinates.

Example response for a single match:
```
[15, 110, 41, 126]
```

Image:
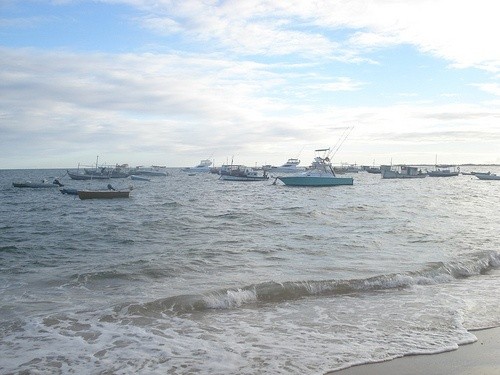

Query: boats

[78, 184, 130, 200]
[60, 187, 80, 195]
[184, 159, 500, 184]
[274, 125, 358, 187]
[66, 154, 169, 181]
[12, 180, 64, 188]
